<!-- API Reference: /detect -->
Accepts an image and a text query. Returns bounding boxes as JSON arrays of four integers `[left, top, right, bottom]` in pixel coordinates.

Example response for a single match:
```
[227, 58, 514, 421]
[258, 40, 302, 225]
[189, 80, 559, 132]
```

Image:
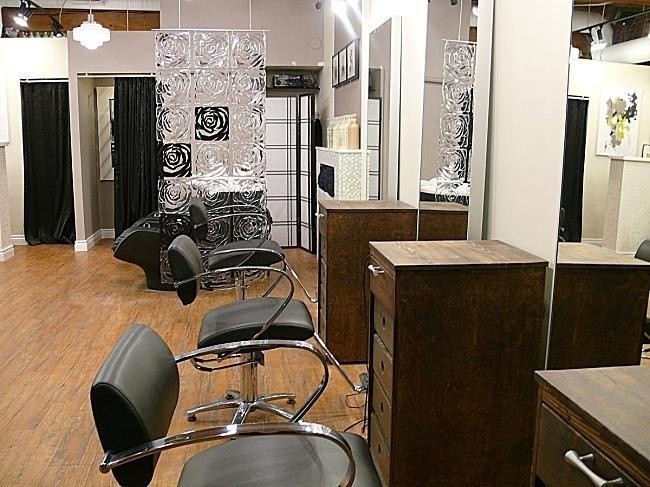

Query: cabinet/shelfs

[367, 238, 549, 487]
[547, 242, 650, 369]
[528, 364, 650, 487]
[317, 200, 418, 366]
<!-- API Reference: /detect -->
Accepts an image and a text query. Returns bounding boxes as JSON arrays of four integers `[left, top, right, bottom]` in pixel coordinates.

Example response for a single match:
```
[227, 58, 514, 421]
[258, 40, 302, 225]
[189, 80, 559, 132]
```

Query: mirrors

[366, 13, 402, 200]
[419, 0, 495, 241]
[550, 0, 649, 368]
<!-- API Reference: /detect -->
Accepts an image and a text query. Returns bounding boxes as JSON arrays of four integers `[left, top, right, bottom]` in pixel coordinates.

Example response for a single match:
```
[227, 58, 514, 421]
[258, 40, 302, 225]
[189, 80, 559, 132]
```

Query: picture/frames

[332, 39, 359, 88]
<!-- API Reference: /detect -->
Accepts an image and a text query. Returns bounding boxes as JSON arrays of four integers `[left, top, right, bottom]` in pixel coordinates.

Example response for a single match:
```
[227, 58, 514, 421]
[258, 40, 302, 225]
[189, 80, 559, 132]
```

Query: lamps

[13, 0, 111, 51]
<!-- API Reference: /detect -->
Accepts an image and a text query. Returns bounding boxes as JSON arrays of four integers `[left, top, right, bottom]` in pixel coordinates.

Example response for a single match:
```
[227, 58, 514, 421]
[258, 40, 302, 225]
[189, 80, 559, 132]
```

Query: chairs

[91, 322, 382, 487]
[186, 197, 320, 305]
[165, 234, 367, 440]
